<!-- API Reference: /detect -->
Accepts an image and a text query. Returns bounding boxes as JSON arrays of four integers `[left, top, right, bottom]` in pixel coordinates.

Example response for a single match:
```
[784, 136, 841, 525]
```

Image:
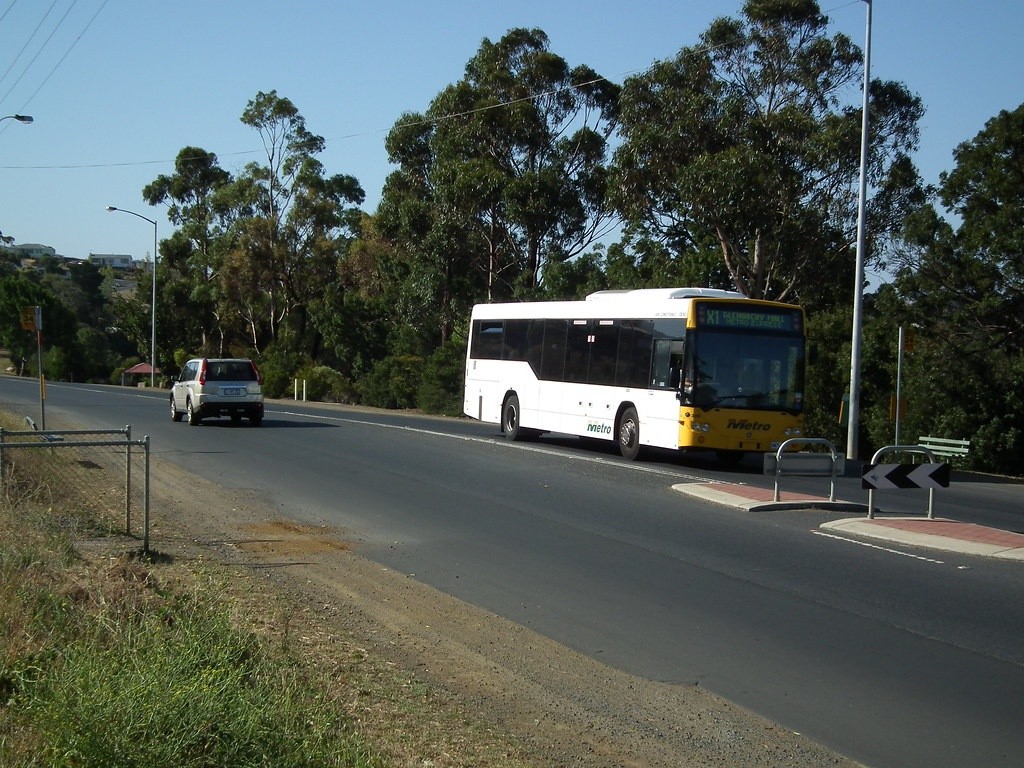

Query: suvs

[170, 357, 265, 426]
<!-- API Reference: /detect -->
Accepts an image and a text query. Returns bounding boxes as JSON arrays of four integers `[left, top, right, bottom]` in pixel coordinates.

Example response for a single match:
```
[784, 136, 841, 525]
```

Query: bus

[462, 286, 818, 463]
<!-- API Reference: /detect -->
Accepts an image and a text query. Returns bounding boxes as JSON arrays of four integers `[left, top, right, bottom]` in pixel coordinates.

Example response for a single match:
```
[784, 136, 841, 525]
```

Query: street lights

[105, 205, 159, 389]
[1, 114, 33, 125]
